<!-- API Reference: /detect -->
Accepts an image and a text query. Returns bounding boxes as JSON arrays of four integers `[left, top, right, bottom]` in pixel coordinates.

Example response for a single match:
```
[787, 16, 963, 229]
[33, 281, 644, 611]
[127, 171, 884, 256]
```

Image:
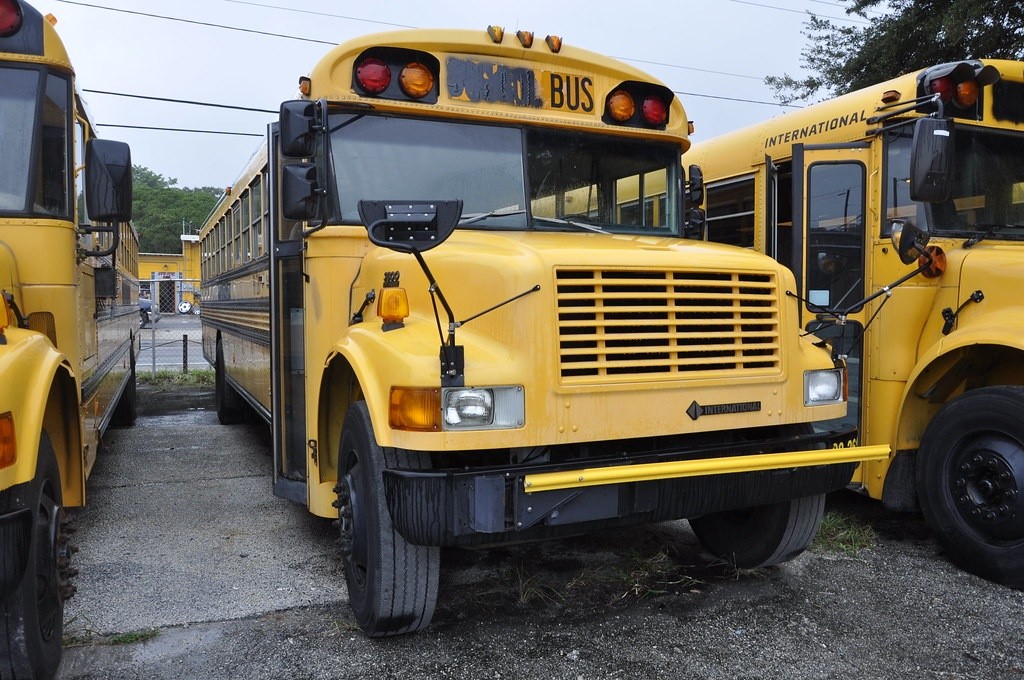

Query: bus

[203, 24, 893, 640]
[2, 1, 140, 680]
[498, 57, 1024, 594]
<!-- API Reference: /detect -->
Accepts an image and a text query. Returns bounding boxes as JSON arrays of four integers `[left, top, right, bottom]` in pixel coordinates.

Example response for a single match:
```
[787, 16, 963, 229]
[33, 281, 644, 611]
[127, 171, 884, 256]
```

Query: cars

[140, 297, 152, 329]
